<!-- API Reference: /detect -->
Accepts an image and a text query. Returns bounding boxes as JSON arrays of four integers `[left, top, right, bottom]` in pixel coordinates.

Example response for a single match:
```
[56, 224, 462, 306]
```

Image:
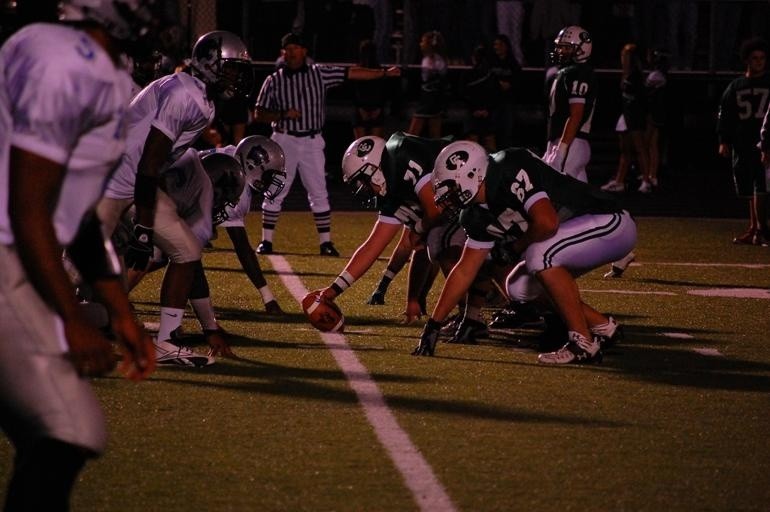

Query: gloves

[368, 291, 384, 305]
[550, 148, 565, 172]
[411, 313, 486, 356]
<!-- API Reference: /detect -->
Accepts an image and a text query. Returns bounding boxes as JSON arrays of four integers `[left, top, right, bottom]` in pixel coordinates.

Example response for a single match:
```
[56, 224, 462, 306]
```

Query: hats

[282, 31, 310, 47]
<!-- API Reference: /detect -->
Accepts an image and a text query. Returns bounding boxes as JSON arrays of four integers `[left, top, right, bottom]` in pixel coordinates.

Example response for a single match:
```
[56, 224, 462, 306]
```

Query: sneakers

[601, 180, 625, 192]
[605, 252, 635, 278]
[319, 241, 339, 256]
[153, 329, 215, 368]
[638, 174, 658, 193]
[255, 240, 272, 254]
[488, 303, 622, 365]
[733, 229, 770, 245]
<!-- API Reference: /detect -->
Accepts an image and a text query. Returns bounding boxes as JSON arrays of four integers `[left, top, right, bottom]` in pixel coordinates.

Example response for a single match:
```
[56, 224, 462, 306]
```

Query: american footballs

[302, 293, 345, 333]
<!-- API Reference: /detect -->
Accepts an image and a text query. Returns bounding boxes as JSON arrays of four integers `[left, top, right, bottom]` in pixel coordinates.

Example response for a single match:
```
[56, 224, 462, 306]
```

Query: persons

[716, 37, 768, 245]
[248, 4, 323, 65]
[757, 104, 770, 196]
[0, 0, 155, 512]
[253, 34, 400, 256]
[63, 0, 283, 378]
[326, 1, 709, 364]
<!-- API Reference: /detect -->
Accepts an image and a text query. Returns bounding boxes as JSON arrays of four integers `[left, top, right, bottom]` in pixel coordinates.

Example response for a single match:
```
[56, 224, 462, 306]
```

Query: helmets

[192, 32, 253, 102]
[59, 0, 153, 51]
[341, 137, 386, 197]
[554, 25, 593, 63]
[430, 141, 491, 208]
[230, 134, 287, 193]
